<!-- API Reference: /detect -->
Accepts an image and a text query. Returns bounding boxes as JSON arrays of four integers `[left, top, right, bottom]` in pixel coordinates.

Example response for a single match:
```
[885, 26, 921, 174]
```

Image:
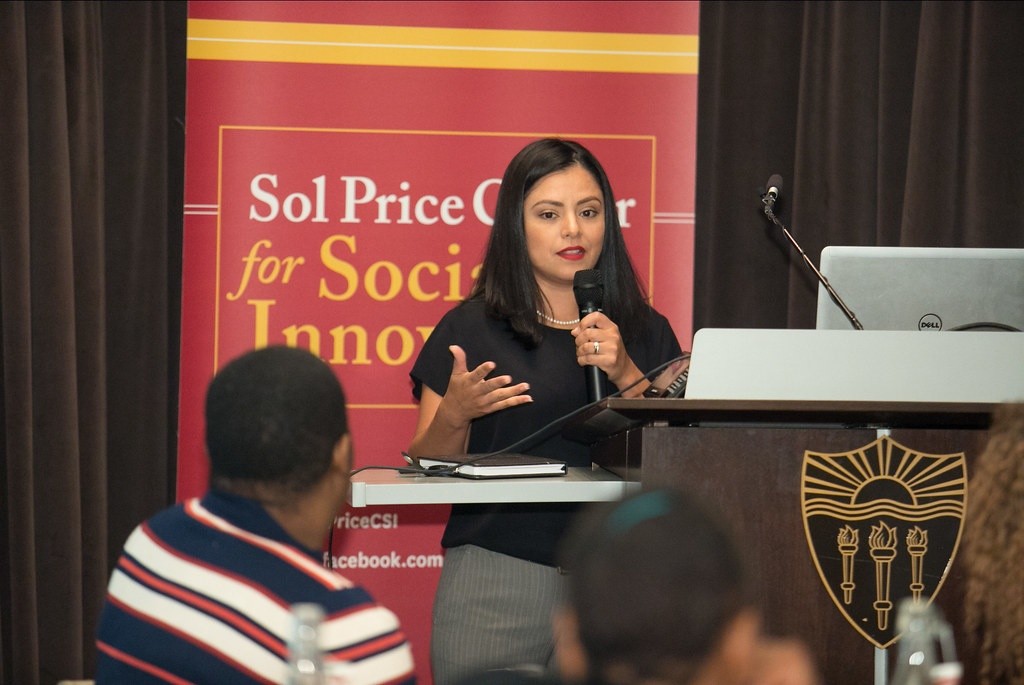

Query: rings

[593, 341, 600, 355]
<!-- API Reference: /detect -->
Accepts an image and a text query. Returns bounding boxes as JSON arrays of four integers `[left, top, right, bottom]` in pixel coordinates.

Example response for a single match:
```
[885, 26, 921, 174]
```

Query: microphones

[764, 173, 784, 207]
[572, 268, 610, 404]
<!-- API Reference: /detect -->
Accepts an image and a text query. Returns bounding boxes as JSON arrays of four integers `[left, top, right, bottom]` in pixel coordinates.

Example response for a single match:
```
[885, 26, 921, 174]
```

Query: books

[415, 451, 570, 479]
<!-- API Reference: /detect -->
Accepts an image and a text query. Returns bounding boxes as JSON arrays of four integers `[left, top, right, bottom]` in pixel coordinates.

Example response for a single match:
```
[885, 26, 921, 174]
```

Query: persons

[446, 482, 815, 683]
[410, 138, 685, 684]
[92, 345, 416, 684]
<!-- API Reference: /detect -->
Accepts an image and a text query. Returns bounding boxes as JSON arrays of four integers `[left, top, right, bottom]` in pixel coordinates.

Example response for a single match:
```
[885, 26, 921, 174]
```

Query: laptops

[811, 246, 1024, 332]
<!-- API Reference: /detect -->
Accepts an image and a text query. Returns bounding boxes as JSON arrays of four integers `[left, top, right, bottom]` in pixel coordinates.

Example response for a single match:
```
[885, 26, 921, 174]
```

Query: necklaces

[536, 309, 580, 325]
